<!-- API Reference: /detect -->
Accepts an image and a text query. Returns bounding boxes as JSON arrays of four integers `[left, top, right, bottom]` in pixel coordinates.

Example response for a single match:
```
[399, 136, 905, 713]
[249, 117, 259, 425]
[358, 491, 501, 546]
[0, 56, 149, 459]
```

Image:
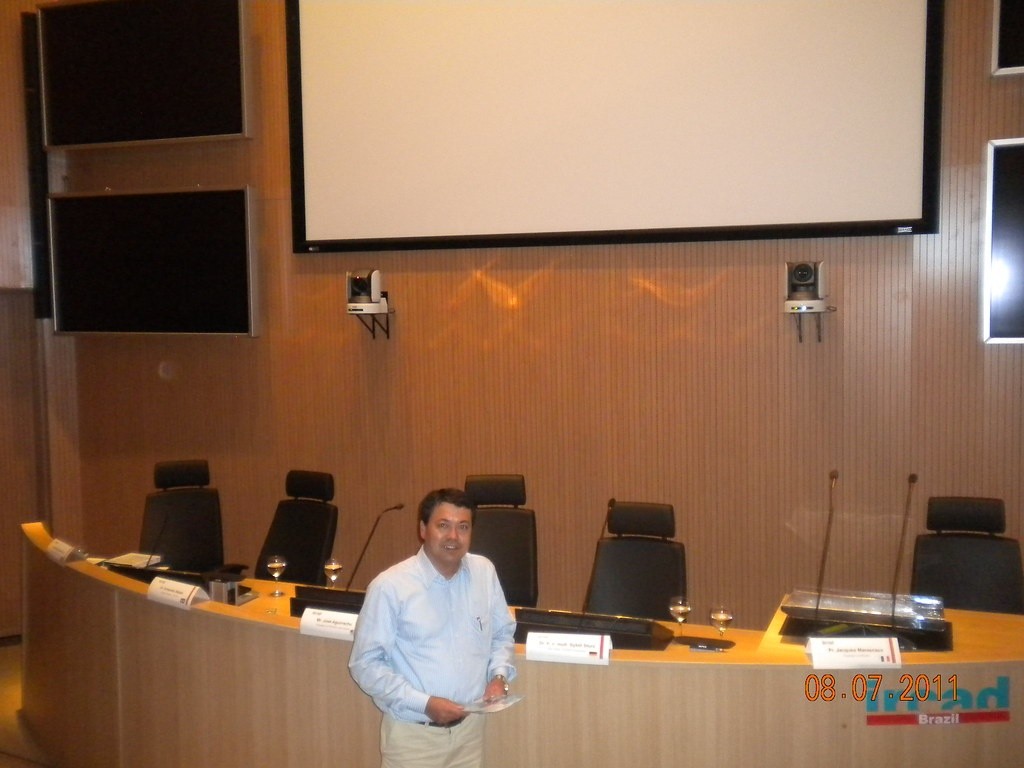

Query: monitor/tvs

[991, 0, 1024, 75]
[37, 0, 255, 151]
[46, 183, 254, 338]
[983, 138, 1024, 343]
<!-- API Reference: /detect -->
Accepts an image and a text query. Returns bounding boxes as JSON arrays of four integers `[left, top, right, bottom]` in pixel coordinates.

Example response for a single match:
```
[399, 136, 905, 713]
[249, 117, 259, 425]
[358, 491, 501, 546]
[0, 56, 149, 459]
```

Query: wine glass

[324, 556, 343, 588]
[710, 605, 733, 654]
[267, 555, 287, 597]
[668, 598, 691, 646]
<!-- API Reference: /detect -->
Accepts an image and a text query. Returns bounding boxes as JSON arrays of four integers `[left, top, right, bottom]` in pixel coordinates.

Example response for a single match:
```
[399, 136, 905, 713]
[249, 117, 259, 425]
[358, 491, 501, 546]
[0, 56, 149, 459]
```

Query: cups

[74, 545, 89, 559]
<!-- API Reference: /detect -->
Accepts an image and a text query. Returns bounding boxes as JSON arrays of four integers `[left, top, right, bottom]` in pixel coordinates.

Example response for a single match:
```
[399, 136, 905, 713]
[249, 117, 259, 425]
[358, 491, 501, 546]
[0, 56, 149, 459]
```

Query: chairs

[460, 471, 537, 608]
[911, 497, 1024, 617]
[583, 498, 689, 619]
[250, 469, 337, 586]
[139, 459, 224, 573]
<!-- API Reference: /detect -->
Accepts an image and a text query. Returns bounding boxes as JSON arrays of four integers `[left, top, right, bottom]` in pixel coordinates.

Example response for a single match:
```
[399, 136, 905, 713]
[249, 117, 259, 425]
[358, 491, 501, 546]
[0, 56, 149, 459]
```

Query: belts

[420, 719, 465, 729]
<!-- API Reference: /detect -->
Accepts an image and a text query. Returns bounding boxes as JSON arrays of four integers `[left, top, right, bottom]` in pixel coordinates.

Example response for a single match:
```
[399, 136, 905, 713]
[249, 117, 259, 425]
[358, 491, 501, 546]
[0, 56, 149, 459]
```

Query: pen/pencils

[690, 645, 724, 652]
[477, 617, 483, 630]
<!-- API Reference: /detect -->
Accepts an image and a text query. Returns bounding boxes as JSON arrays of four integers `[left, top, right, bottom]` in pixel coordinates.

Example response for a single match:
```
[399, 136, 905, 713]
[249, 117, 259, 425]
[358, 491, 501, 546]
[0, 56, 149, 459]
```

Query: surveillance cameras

[783, 261, 829, 313]
[345, 270, 389, 315]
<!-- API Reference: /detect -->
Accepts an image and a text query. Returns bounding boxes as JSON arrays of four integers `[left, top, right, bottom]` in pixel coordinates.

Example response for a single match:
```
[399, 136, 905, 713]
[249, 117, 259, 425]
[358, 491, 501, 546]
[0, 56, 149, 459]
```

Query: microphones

[809, 471, 840, 637]
[347, 503, 404, 590]
[582, 496, 615, 610]
[145, 506, 173, 566]
[890, 473, 918, 631]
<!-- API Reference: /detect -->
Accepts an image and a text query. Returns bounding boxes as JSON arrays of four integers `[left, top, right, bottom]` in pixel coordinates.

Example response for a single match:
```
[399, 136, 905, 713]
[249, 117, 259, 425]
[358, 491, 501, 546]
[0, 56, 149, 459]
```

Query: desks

[13, 521, 1024, 768]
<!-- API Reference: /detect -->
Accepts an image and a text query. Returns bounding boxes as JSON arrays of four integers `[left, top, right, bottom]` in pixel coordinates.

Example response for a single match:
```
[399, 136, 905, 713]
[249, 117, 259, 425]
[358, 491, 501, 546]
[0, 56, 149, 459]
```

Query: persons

[348, 487, 518, 768]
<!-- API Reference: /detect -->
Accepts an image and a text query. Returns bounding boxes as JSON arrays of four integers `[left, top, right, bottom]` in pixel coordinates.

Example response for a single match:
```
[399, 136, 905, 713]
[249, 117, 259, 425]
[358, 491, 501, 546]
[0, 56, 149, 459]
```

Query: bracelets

[495, 674, 510, 698]
